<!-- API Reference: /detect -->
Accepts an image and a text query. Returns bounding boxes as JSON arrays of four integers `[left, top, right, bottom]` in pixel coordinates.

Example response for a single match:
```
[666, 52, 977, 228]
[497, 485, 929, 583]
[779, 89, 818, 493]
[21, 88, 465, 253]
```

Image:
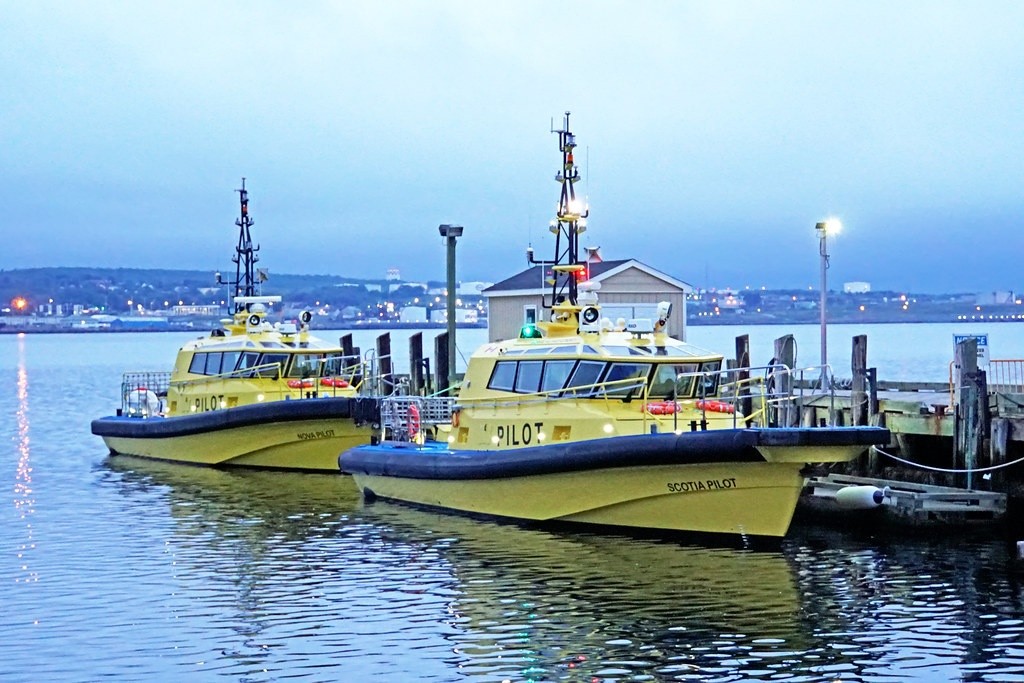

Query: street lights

[438, 224, 464, 396]
[815, 220, 837, 392]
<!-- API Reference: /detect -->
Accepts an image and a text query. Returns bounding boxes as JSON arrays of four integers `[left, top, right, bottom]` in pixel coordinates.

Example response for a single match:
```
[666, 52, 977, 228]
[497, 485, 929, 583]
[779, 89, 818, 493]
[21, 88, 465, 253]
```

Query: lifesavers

[406, 405, 419, 434]
[641, 401, 681, 415]
[696, 400, 735, 413]
[321, 378, 348, 387]
[287, 379, 314, 388]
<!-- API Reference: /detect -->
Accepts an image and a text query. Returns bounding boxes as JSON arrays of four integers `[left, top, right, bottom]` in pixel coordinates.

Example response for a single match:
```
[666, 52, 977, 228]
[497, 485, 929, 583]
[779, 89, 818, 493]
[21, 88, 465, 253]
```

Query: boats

[800, 469, 1011, 536]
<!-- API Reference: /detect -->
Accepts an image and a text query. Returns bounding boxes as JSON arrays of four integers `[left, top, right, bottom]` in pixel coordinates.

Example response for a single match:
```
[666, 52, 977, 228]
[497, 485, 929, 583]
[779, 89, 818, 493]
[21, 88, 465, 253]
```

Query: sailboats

[91, 176, 384, 470]
[330, 104, 892, 541]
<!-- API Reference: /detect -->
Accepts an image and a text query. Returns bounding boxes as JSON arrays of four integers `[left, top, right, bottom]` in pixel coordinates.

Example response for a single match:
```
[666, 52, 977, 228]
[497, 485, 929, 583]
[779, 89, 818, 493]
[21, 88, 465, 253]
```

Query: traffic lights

[521, 324, 538, 338]
[578, 305, 601, 329]
[577, 270, 589, 279]
